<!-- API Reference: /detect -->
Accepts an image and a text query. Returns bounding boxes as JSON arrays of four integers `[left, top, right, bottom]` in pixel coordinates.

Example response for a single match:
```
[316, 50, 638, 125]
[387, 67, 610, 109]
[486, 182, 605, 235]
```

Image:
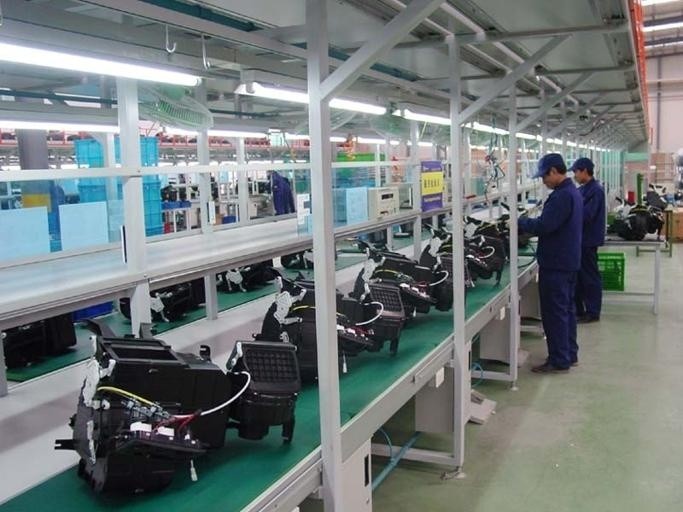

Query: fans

[136, 75, 215, 134]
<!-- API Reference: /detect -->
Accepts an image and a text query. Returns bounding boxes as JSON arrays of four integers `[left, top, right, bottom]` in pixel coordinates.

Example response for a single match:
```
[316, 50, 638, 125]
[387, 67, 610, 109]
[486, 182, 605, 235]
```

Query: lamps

[231, 65, 511, 136]
[0, 35, 205, 91]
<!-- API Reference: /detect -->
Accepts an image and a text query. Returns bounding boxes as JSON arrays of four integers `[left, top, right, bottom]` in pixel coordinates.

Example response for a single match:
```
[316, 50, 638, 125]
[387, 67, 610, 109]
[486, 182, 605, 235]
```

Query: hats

[531, 152, 567, 179]
[568, 157, 594, 171]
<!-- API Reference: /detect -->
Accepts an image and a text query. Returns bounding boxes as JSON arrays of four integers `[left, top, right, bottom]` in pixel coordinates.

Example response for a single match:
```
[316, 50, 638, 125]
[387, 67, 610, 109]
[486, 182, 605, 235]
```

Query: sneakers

[532, 362, 570, 374]
[579, 316, 600, 323]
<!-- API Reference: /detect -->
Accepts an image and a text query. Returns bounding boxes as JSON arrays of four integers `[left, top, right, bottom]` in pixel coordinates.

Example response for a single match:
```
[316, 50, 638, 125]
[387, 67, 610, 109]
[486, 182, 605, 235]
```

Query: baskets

[596, 253, 625, 290]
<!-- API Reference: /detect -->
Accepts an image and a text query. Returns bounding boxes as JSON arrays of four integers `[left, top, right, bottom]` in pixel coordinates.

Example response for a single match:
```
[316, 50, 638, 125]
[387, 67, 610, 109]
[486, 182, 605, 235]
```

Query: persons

[516, 152, 583, 375]
[566, 158, 605, 324]
[263, 170, 295, 216]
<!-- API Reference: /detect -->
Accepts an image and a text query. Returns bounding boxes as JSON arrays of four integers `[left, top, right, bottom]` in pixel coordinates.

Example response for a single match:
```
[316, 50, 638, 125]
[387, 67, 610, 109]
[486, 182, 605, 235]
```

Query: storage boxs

[74, 136, 166, 243]
